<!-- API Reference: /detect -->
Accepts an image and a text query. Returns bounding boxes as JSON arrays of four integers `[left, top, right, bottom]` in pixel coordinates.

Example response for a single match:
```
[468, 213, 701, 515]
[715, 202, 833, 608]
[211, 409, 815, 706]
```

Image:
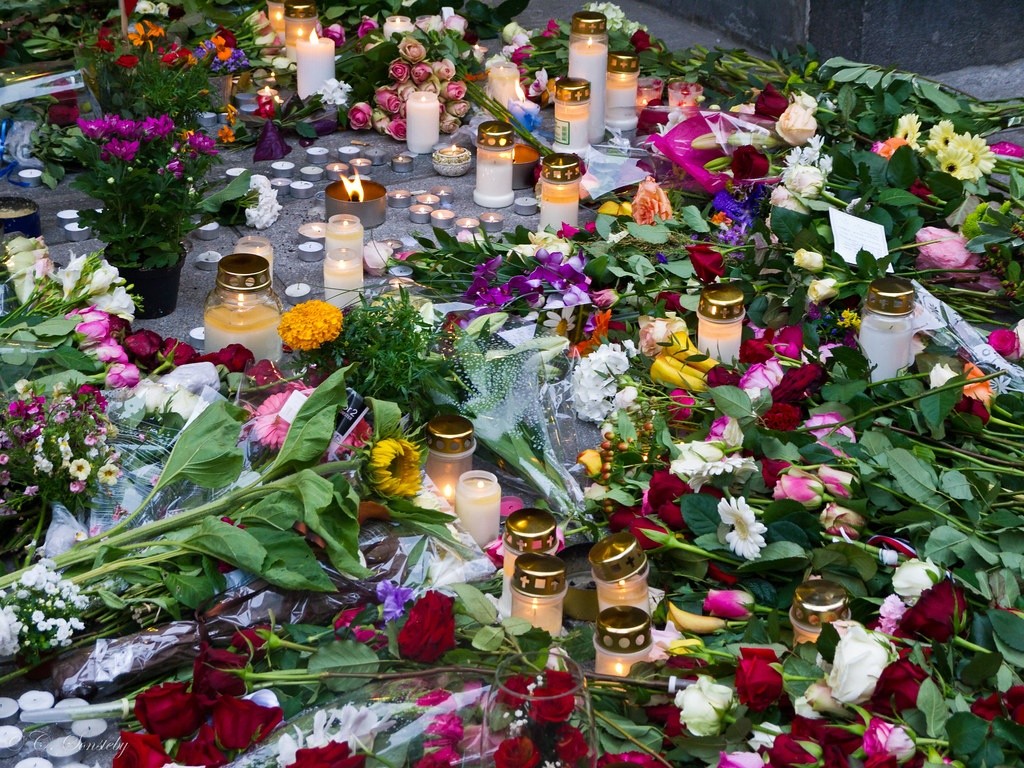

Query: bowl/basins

[432, 146, 471, 176]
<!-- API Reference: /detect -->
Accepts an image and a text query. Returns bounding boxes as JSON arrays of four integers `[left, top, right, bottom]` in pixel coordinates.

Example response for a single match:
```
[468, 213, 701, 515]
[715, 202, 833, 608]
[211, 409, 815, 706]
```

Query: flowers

[0, 0, 1024, 767]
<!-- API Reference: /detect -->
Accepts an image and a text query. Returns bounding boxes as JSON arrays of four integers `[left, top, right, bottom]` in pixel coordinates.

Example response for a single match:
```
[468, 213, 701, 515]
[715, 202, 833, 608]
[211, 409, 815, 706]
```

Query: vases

[103, 242, 188, 321]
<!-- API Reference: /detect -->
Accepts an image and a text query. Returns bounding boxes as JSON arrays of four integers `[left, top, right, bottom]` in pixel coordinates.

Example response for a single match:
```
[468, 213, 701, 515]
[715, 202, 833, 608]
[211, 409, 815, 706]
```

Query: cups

[635, 75, 665, 110]
[667, 80, 704, 108]
[479, 650, 598, 767]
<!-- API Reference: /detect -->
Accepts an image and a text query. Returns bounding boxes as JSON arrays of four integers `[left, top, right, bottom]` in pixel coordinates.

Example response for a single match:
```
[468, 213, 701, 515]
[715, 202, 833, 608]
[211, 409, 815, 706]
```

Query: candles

[18, 0, 918, 702]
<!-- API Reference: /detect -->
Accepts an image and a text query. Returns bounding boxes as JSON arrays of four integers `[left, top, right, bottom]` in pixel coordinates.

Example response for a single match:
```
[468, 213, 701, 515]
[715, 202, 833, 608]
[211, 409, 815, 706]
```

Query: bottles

[860, 277, 913, 380]
[490, 62, 520, 108]
[405, 91, 440, 154]
[538, 153, 581, 235]
[789, 579, 851, 647]
[694, 283, 744, 372]
[325, 214, 363, 256]
[423, 411, 657, 685]
[383, 16, 413, 41]
[265, 0, 317, 62]
[473, 121, 515, 209]
[552, 11, 640, 156]
[202, 253, 283, 367]
[234, 236, 275, 287]
[324, 248, 364, 307]
